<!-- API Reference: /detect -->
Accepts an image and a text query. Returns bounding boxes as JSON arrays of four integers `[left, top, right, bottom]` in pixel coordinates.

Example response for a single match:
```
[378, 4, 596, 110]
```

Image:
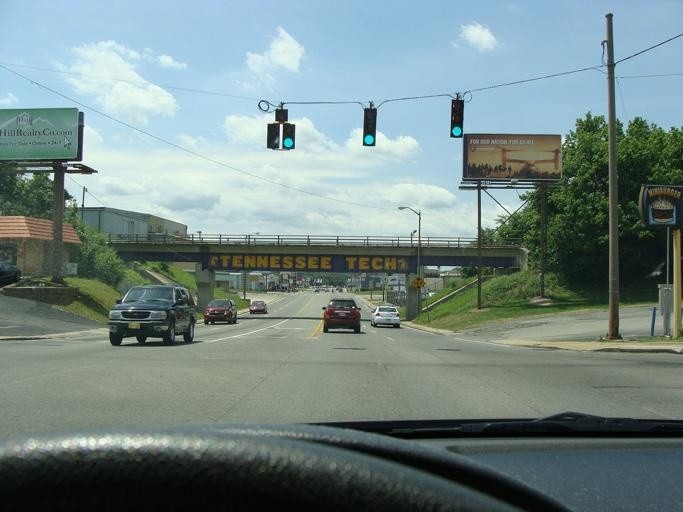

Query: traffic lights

[451, 99, 464, 138]
[267, 122, 295, 150]
[363, 108, 377, 146]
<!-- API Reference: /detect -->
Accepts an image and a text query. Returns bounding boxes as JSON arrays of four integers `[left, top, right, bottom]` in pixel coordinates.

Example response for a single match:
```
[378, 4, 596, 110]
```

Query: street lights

[399, 206, 422, 316]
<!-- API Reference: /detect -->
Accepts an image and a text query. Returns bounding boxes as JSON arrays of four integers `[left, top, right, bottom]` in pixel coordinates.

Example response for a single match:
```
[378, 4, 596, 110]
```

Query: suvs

[108, 284, 197, 345]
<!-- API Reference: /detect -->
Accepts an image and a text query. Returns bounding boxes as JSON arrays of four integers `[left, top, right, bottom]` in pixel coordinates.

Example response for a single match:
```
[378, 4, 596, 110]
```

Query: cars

[321, 298, 362, 333]
[371, 306, 400, 328]
[249, 300, 267, 314]
[204, 299, 236, 324]
